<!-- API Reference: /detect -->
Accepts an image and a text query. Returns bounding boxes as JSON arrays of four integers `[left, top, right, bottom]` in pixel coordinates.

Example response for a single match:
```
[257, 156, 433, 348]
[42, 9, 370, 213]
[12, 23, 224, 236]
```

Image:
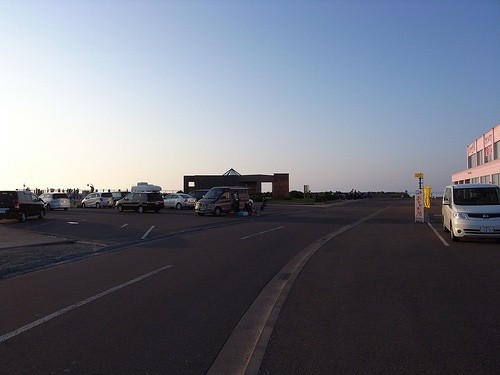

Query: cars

[115, 192, 164, 213]
[110, 191, 131, 202]
[162, 193, 197, 209]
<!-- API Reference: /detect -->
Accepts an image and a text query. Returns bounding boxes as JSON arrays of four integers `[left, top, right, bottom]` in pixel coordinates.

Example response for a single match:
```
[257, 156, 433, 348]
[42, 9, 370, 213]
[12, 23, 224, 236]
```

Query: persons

[243, 201, 253, 215]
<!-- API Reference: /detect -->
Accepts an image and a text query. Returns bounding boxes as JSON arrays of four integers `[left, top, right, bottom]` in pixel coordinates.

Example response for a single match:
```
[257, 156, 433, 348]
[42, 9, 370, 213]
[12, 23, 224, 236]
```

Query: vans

[441, 184, 500, 241]
[38, 192, 71, 210]
[195, 186, 250, 216]
[0, 190, 46, 222]
[81, 192, 113, 209]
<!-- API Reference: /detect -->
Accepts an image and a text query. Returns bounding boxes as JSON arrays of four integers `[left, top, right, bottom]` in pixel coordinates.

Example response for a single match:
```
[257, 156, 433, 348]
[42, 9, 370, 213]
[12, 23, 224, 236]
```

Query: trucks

[131, 182, 162, 192]
